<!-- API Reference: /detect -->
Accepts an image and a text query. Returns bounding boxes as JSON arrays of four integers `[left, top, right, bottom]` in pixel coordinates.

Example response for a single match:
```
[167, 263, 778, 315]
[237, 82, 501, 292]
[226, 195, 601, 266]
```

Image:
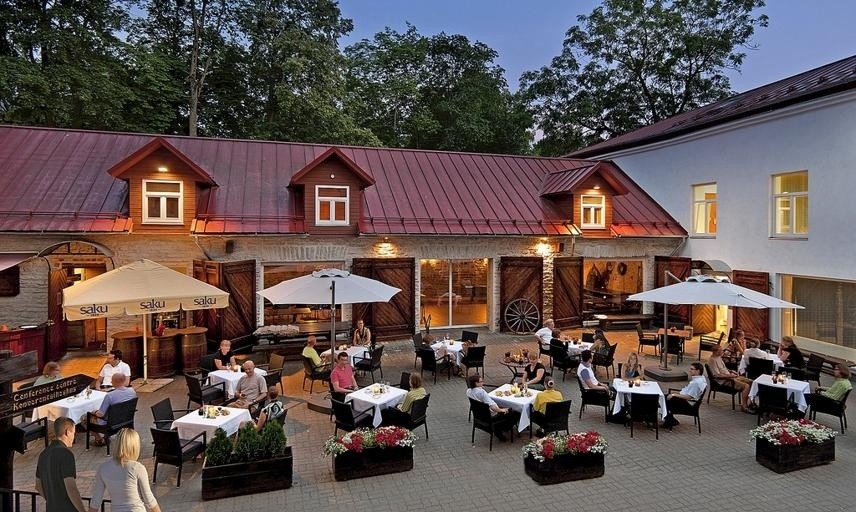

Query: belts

[102, 385, 113, 388]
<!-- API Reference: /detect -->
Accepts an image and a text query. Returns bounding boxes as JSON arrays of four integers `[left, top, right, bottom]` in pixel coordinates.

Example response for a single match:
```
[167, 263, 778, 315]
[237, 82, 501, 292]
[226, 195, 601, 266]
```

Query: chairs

[17, 382, 36, 423]
[150, 397, 194, 457]
[261, 371, 279, 388]
[258, 410, 288, 436]
[221, 376, 272, 419]
[183, 371, 226, 414]
[85, 397, 139, 456]
[13, 412, 49, 453]
[197, 354, 218, 385]
[151, 427, 207, 487]
[254, 353, 285, 395]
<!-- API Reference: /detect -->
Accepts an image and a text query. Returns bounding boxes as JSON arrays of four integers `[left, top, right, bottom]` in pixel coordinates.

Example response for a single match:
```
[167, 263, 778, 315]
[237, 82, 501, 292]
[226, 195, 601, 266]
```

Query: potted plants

[200, 419, 293, 501]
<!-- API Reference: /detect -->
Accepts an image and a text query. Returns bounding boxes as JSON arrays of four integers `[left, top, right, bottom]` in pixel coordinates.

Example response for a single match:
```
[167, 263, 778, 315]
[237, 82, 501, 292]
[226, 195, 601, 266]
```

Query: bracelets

[353, 385, 358, 388]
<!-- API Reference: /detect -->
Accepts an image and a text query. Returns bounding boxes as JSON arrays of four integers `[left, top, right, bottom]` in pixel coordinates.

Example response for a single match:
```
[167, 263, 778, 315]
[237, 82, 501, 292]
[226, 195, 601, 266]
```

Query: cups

[233, 364, 238, 372]
[371, 380, 390, 399]
[199, 404, 215, 418]
[511, 378, 528, 397]
[766, 350, 770, 356]
[771, 371, 792, 384]
[335, 342, 352, 350]
[629, 376, 641, 387]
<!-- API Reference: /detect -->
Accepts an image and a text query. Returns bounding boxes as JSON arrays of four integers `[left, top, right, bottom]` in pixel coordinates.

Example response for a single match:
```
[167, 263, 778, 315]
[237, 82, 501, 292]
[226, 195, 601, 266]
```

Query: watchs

[80, 373, 137, 446]
[252, 399, 257, 404]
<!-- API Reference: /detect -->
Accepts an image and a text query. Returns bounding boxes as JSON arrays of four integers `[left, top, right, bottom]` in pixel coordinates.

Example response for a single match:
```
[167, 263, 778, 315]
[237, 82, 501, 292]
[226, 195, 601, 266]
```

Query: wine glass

[446, 333, 450, 340]
[436, 337, 440, 343]
[226, 362, 232, 373]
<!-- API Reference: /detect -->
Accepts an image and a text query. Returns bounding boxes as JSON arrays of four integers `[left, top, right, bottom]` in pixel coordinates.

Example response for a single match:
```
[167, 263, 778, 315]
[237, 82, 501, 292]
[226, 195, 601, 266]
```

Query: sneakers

[90, 438, 107, 447]
[658, 423, 671, 430]
[668, 419, 680, 426]
[741, 405, 756, 415]
[748, 403, 759, 411]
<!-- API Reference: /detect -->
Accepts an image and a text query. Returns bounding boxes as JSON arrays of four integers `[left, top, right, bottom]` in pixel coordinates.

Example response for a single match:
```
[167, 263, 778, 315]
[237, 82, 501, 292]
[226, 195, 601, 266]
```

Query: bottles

[238, 366, 242, 373]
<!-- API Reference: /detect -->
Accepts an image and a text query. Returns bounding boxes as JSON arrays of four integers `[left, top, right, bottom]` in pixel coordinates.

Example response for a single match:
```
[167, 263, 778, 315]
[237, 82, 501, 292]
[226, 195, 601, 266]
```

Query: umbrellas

[61, 258, 231, 392]
[625, 269, 806, 382]
[255, 267, 404, 352]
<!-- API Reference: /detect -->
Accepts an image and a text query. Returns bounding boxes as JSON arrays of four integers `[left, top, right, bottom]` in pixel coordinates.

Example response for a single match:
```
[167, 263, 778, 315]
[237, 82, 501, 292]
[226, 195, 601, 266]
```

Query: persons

[708, 330, 767, 413]
[803, 363, 851, 406]
[302, 336, 332, 372]
[396, 372, 427, 413]
[330, 352, 360, 393]
[35, 417, 86, 512]
[88, 427, 161, 512]
[95, 350, 131, 391]
[226, 360, 268, 414]
[535, 319, 576, 373]
[466, 375, 521, 441]
[522, 351, 563, 436]
[232, 386, 283, 453]
[778, 336, 805, 379]
[420, 334, 456, 366]
[33, 361, 63, 387]
[352, 320, 371, 346]
[621, 352, 644, 381]
[578, 330, 616, 401]
[662, 363, 706, 428]
[211, 340, 236, 371]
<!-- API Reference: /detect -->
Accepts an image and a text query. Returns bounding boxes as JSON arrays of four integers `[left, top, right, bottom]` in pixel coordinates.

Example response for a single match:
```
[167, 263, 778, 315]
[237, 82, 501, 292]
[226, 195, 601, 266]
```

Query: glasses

[690, 367, 699, 371]
[477, 380, 485, 384]
[834, 368, 841, 371]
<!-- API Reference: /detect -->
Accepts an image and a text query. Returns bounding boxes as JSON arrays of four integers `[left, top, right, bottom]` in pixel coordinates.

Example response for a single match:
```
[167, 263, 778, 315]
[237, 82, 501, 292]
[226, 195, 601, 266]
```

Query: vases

[525, 452, 603, 485]
[332, 446, 413, 482]
[756, 435, 835, 474]
